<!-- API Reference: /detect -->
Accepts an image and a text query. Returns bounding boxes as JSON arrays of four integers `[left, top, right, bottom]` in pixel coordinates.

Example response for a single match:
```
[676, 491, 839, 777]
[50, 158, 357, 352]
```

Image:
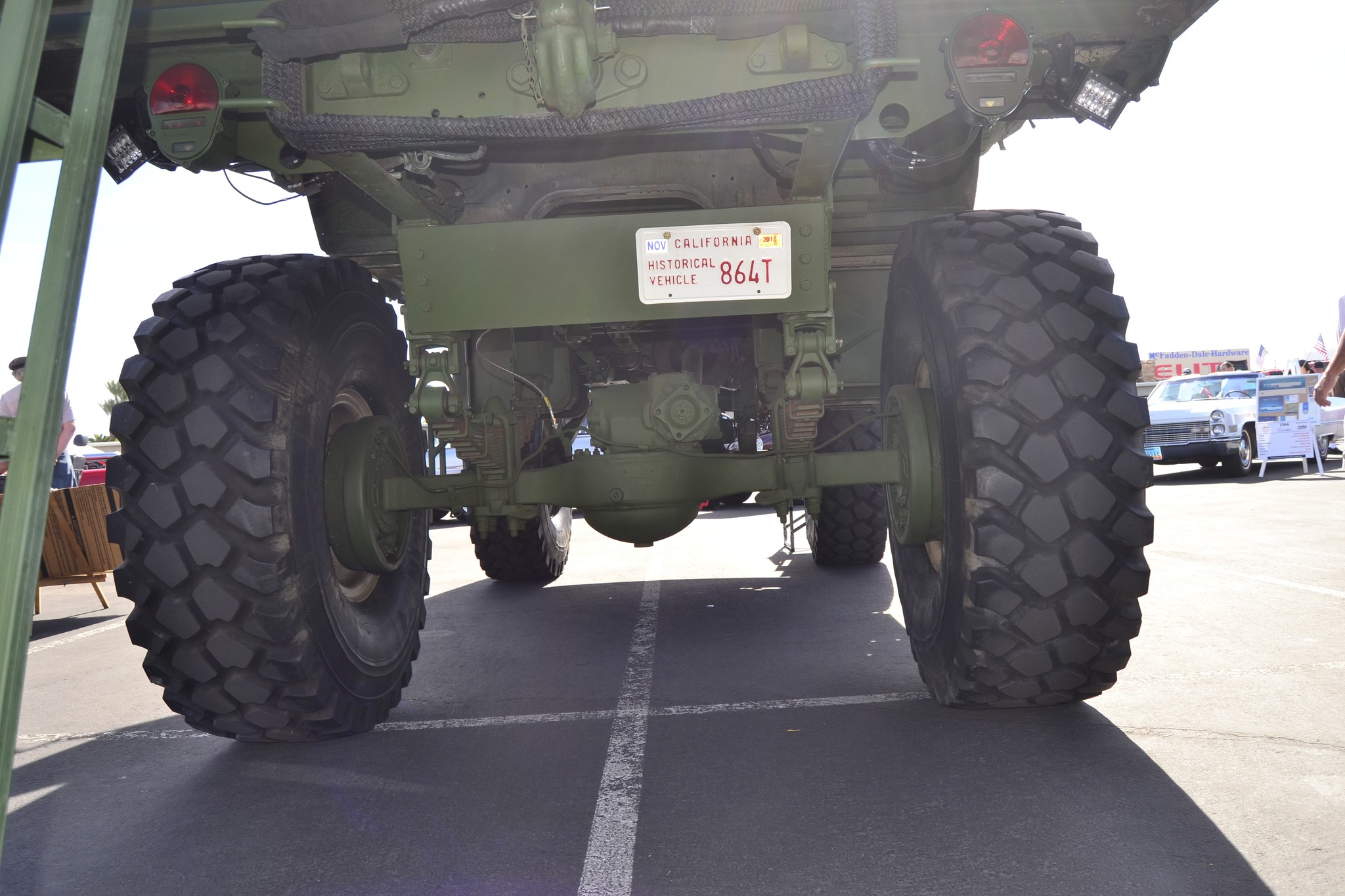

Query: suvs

[1144, 370, 1268, 476]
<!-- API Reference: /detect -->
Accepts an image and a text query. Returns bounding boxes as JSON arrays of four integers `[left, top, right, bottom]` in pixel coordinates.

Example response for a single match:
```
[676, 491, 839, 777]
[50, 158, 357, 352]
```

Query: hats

[1181, 368, 1191, 375]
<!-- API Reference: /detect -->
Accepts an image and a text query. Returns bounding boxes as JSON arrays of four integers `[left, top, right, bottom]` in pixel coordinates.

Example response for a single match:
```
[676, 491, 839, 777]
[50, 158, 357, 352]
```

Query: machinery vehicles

[0, 0, 1217, 896]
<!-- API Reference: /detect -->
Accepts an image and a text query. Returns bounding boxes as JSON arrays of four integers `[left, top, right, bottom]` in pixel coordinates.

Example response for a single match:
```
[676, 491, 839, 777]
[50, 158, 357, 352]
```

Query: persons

[1177, 368, 1208, 401]
[1299, 360, 1314, 374]
[1312, 326, 1345, 470]
[1218, 362, 1247, 398]
[1312, 361, 1343, 455]
[0, 356, 80, 489]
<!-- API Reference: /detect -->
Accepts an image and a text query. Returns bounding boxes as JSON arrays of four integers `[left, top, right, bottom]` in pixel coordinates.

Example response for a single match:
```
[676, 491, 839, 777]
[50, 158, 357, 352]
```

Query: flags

[1314, 334, 1328, 360]
[1255, 345, 1269, 367]
